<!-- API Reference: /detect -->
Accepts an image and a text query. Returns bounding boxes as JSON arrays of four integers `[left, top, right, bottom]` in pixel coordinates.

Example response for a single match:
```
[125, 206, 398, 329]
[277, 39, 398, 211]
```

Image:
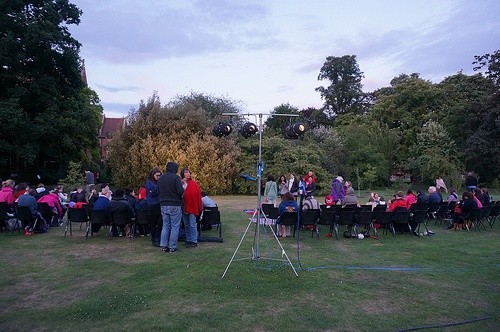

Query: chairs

[16, 206, 43, 233]
[63, 203, 222, 243]
[36, 202, 59, 227]
[0, 201, 21, 236]
[259, 201, 500, 241]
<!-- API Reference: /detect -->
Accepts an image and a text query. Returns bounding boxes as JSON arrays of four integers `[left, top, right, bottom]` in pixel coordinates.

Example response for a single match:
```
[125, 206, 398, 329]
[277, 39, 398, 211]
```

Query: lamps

[239, 115, 258, 139]
[283, 116, 306, 141]
[212, 115, 234, 138]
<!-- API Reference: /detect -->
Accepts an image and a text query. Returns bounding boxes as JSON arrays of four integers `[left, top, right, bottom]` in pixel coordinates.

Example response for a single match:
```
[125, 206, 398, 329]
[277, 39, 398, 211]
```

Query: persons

[146, 168, 163, 247]
[276, 192, 298, 236]
[331, 175, 344, 205]
[465, 172, 478, 193]
[302, 194, 321, 237]
[287, 173, 299, 199]
[263, 170, 491, 238]
[0, 179, 217, 237]
[155, 162, 184, 252]
[84, 169, 95, 194]
[178, 168, 203, 247]
[278, 175, 289, 199]
[264, 176, 277, 207]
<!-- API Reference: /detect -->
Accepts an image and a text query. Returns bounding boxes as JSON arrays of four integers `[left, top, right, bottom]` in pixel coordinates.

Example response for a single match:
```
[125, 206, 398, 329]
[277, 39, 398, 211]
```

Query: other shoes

[162, 247, 175, 252]
[183, 241, 197, 248]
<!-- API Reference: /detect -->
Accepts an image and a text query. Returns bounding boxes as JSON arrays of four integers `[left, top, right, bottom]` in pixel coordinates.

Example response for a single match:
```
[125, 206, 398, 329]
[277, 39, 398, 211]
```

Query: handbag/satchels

[7, 218, 22, 230]
[264, 195, 269, 201]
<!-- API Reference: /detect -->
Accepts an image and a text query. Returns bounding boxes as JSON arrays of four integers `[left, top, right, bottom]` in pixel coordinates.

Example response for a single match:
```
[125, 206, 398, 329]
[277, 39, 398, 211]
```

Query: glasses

[154, 173, 161, 176]
